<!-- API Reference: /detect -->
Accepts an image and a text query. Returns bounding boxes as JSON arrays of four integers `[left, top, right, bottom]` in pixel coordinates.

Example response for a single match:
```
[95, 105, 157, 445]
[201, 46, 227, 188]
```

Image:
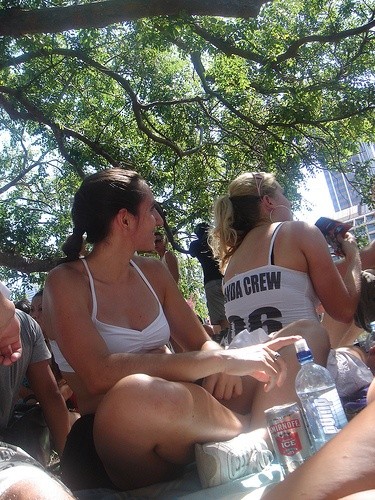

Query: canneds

[264, 402, 313, 479]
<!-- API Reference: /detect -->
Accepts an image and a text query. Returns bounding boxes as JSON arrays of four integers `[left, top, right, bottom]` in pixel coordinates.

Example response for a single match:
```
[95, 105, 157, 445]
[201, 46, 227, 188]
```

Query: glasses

[252, 172, 263, 201]
[155, 238, 163, 244]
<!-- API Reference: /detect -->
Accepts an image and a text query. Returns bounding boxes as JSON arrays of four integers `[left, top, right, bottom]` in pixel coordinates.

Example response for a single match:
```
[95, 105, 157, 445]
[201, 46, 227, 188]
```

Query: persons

[0, 281, 81, 500]
[154, 231, 179, 284]
[206, 172, 375, 500]
[43, 169, 331, 492]
[189, 222, 228, 334]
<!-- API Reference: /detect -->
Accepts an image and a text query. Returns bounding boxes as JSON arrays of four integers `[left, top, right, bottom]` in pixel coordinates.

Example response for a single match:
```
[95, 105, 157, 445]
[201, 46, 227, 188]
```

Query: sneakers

[195, 433, 274, 489]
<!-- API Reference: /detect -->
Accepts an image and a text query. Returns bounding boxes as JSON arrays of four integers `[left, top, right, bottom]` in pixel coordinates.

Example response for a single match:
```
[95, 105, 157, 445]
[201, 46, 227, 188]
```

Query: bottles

[293, 335, 349, 453]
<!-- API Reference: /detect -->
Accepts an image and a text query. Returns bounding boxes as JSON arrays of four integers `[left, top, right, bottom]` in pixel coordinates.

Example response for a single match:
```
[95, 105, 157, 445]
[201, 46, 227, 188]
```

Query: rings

[274, 352, 281, 360]
